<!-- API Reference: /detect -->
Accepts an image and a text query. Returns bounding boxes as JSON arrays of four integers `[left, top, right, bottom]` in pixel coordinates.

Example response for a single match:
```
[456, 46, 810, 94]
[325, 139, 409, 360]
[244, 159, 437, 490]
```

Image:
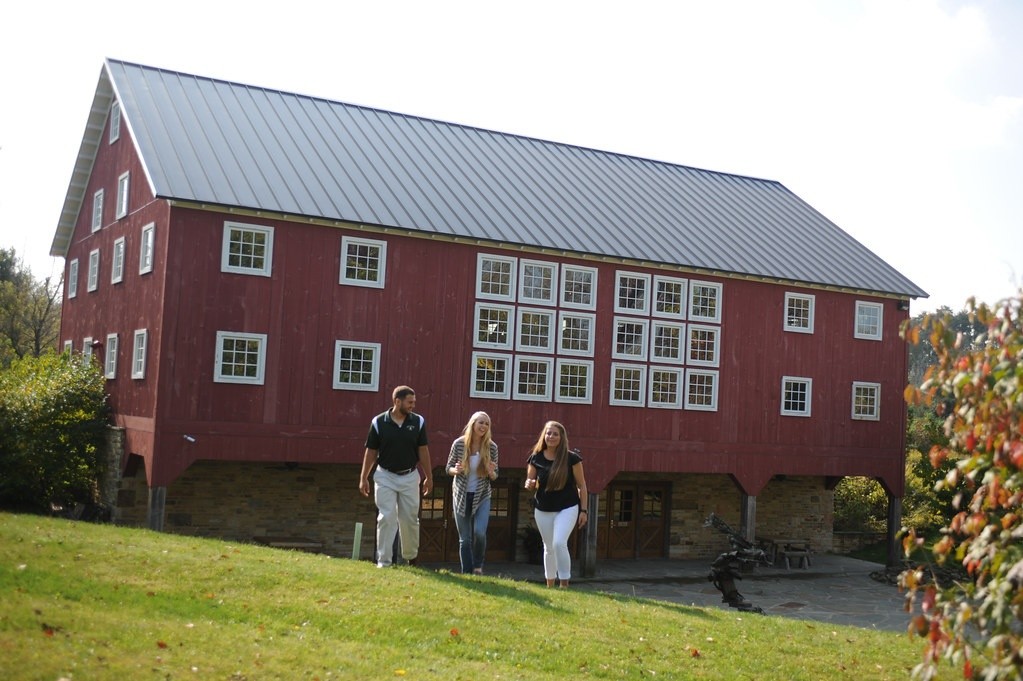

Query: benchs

[784, 545, 818, 564]
[779, 551, 808, 570]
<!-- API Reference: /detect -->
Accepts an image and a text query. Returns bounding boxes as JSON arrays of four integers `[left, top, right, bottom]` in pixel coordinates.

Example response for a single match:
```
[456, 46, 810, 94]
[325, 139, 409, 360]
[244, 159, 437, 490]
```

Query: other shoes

[474, 567, 482, 575]
[408, 555, 420, 566]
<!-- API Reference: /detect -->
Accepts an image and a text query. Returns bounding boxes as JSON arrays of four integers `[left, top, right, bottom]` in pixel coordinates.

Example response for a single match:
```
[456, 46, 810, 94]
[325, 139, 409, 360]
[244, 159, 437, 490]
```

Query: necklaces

[399, 425, 401, 428]
[476, 452, 479, 454]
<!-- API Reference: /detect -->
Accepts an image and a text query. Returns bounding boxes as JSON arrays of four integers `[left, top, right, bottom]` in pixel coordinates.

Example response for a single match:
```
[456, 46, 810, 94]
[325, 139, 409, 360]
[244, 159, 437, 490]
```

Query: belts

[380, 463, 416, 475]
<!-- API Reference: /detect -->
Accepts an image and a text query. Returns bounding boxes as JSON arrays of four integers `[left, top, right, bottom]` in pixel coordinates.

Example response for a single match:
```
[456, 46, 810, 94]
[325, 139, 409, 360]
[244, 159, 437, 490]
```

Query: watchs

[580, 510, 588, 514]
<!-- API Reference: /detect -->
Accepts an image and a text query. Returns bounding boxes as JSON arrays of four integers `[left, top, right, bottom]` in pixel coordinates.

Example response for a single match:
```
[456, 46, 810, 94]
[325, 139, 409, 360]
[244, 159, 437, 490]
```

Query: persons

[445, 411, 499, 576]
[359, 386, 433, 568]
[525, 421, 588, 588]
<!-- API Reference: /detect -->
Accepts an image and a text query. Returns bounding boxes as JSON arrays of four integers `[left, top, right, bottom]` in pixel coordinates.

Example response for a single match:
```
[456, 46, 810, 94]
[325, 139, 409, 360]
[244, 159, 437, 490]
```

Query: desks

[253, 536, 323, 551]
[754, 535, 813, 568]
